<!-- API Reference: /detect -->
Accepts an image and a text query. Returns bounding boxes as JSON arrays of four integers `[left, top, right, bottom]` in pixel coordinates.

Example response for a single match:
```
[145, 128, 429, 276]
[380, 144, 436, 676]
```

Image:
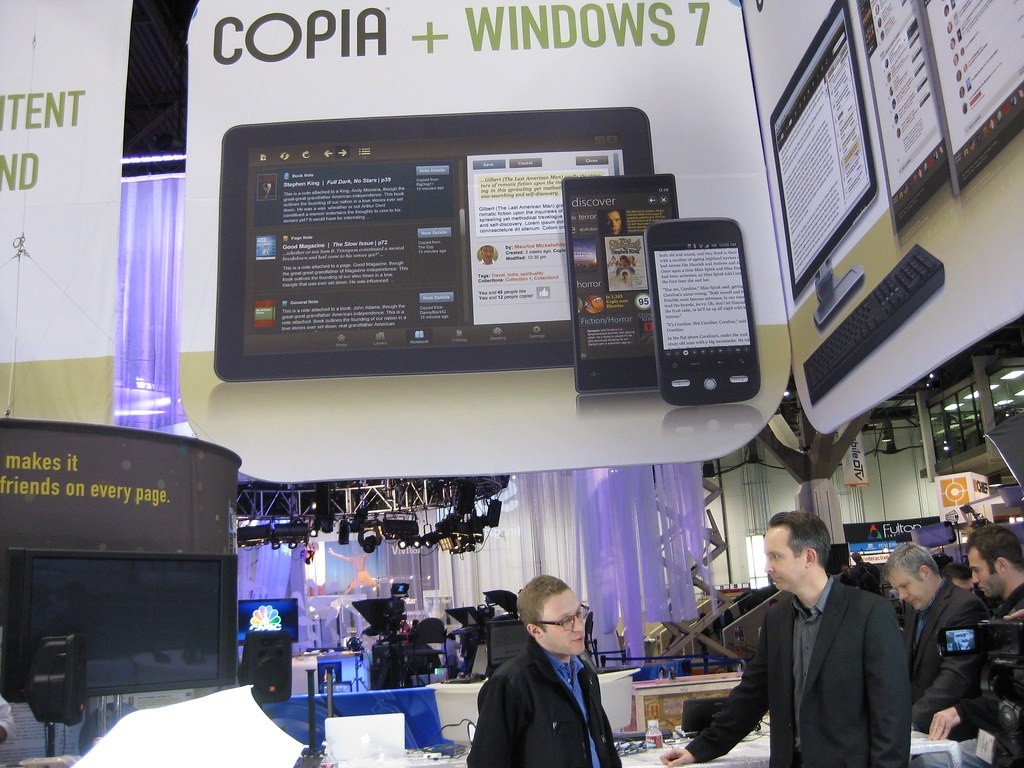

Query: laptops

[674, 696, 729, 738]
[612, 728, 672, 742]
[325, 713, 405, 760]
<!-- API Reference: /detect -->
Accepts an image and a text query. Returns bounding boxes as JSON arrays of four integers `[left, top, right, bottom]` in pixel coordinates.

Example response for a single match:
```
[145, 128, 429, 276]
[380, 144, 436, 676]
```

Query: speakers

[237, 631, 293, 703]
[24, 633, 87, 727]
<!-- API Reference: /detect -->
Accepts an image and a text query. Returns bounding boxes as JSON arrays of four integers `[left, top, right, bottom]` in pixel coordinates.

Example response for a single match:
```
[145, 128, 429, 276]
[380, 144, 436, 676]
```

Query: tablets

[214, 105, 654, 382]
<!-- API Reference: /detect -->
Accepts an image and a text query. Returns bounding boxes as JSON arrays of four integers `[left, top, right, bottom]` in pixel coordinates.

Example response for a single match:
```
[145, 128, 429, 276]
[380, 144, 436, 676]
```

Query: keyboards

[802, 242, 946, 403]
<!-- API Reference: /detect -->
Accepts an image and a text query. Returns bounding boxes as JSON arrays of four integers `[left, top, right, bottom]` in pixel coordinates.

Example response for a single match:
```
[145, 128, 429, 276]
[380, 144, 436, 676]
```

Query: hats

[852, 553, 861, 562]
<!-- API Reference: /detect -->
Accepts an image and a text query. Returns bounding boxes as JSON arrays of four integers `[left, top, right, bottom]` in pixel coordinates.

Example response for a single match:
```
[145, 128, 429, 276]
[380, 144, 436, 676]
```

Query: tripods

[374, 627, 425, 690]
[350, 657, 369, 692]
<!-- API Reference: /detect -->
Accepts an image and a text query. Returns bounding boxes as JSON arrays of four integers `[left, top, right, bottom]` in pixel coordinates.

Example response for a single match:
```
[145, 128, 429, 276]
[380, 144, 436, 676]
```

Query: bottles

[646, 718, 663, 753]
[320, 751, 338, 768]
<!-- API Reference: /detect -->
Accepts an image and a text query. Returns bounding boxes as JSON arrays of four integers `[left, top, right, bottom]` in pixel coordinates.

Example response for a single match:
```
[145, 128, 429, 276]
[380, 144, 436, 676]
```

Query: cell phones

[565, 175, 678, 392]
[643, 218, 761, 406]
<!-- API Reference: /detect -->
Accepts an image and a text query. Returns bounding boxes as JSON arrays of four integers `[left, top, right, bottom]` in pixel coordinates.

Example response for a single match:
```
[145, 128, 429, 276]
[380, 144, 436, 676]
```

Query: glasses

[531, 604, 589, 631]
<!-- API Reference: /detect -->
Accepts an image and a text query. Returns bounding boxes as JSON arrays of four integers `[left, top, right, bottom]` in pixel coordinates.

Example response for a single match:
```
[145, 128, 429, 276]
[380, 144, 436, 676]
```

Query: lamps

[882, 429, 893, 442]
[236, 477, 502, 564]
[352, 588, 520, 644]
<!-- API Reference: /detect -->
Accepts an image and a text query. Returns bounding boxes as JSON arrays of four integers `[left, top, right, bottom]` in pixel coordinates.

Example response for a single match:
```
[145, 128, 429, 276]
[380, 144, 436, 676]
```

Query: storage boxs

[434, 667, 640, 742]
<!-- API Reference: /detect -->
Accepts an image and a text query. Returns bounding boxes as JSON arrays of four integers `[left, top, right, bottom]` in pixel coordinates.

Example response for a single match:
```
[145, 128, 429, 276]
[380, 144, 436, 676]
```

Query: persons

[841, 523, 1024, 768]
[0, 695, 14, 746]
[596, 207, 628, 292]
[458, 632, 477, 678]
[401, 619, 419, 644]
[442, 634, 462, 672]
[660, 509, 912, 768]
[329, 546, 380, 596]
[466, 574, 623, 768]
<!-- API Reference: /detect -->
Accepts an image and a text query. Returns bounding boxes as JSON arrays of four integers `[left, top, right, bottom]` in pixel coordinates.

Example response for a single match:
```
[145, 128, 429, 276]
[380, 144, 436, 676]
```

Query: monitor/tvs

[769, 1, 1024, 330]
[238, 597, 298, 646]
[486, 620, 531, 667]
[3, 548, 239, 702]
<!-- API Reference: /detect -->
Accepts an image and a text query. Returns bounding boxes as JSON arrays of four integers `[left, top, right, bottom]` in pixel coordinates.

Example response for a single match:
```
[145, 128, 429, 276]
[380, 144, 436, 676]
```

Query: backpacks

[858, 564, 878, 591]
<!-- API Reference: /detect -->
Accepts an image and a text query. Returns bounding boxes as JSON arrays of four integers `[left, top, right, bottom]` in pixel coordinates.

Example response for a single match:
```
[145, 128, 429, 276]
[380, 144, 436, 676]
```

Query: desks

[407, 721, 963, 768]
